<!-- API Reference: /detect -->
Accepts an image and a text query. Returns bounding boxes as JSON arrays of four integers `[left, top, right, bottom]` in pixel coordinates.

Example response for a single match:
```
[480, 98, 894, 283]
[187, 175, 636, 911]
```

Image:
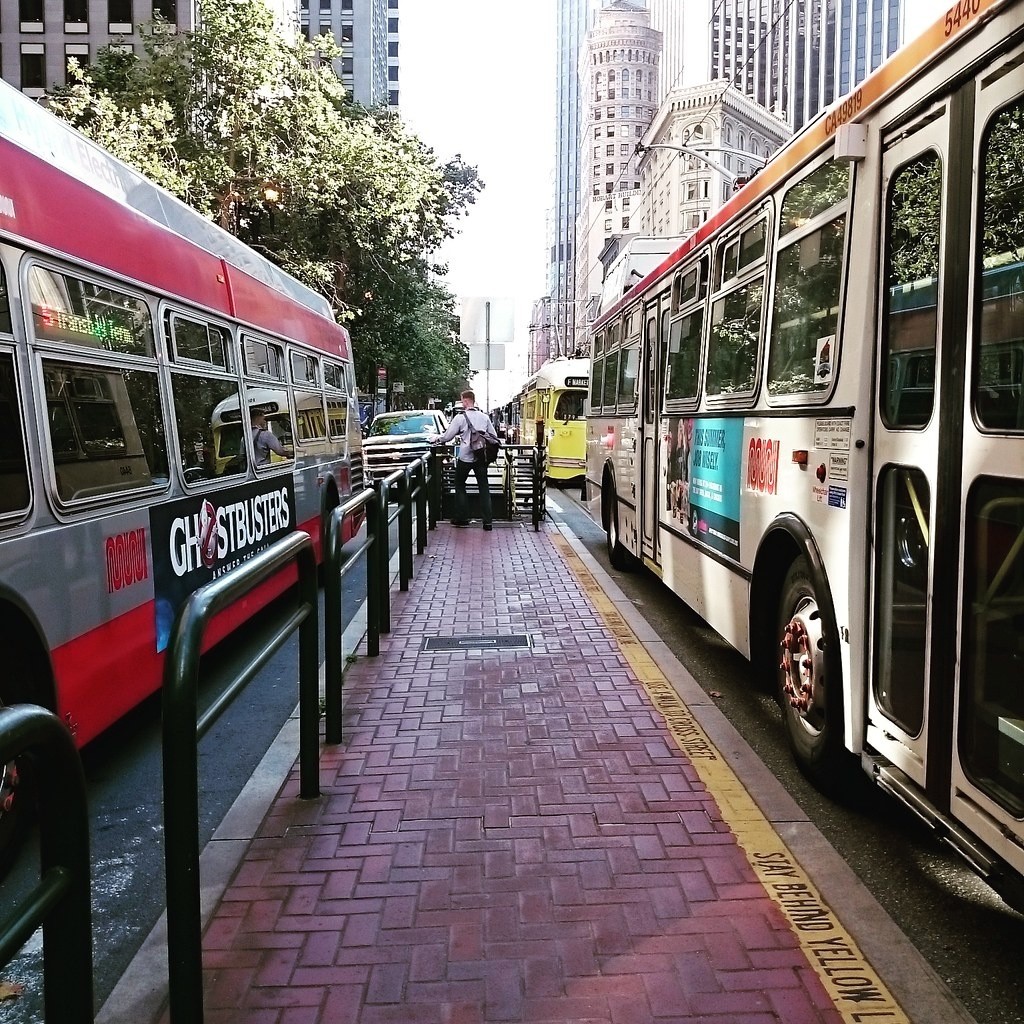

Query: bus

[580, 0, 1024, 922]
[1, 71, 363, 856]
[2, 357, 361, 499]
[487, 357, 590, 489]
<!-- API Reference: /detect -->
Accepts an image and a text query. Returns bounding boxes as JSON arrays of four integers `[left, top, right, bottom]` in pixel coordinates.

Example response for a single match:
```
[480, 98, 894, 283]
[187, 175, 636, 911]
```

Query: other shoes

[450, 519, 468, 527]
[482, 522, 492, 531]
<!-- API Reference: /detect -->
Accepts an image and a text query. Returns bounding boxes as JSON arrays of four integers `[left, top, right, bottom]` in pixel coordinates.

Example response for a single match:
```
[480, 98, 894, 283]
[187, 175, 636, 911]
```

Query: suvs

[362, 409, 452, 490]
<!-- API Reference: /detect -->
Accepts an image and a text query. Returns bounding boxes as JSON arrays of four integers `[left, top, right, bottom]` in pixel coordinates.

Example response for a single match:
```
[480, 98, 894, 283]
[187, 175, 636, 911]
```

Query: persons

[669, 420, 693, 520]
[431, 391, 500, 531]
[240, 409, 293, 467]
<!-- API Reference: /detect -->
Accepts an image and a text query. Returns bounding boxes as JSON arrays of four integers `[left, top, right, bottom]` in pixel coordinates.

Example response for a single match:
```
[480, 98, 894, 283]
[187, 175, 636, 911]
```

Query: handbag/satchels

[464, 412, 499, 463]
[222, 456, 245, 475]
